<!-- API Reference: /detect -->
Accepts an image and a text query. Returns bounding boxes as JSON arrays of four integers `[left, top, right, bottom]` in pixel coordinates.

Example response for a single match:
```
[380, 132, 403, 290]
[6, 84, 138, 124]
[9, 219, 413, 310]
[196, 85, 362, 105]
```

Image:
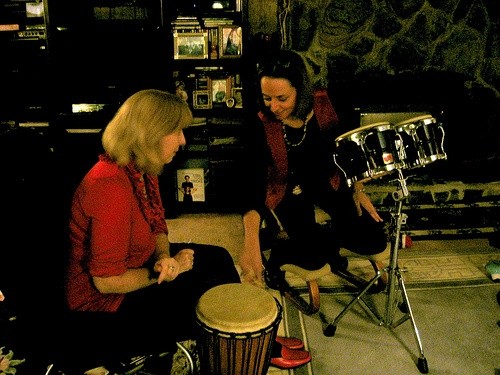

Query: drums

[194, 283, 283, 375]
[335, 122, 404, 184]
[394, 114, 447, 170]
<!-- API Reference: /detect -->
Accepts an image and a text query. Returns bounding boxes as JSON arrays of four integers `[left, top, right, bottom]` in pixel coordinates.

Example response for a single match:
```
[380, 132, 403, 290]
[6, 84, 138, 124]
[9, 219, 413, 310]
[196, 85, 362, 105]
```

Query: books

[171, 16, 234, 46]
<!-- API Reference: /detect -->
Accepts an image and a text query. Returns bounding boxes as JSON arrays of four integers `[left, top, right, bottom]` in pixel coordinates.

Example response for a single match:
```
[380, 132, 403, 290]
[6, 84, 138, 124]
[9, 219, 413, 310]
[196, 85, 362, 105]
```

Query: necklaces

[282, 116, 308, 147]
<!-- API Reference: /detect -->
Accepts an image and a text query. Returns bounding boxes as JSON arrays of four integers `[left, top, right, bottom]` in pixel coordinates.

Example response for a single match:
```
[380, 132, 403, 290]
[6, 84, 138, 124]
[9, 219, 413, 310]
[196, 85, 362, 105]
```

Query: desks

[0, 96, 209, 140]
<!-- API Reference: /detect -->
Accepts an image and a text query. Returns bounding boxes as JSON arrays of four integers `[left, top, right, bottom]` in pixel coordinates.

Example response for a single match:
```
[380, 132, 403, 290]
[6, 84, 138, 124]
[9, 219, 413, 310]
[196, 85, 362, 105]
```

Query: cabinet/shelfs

[0, 0, 52, 98]
[160, 0, 251, 207]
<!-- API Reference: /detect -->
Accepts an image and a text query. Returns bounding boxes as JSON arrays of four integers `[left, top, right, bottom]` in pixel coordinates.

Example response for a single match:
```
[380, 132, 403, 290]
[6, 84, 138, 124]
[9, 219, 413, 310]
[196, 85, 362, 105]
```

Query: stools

[257, 215, 391, 314]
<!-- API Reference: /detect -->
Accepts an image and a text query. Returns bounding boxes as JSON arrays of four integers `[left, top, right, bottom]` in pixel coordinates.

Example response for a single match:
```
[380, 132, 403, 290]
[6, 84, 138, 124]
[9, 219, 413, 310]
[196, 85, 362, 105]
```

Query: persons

[228, 99, 233, 107]
[238, 49, 390, 288]
[178, 174, 197, 201]
[235, 89, 242, 106]
[65, 89, 311, 370]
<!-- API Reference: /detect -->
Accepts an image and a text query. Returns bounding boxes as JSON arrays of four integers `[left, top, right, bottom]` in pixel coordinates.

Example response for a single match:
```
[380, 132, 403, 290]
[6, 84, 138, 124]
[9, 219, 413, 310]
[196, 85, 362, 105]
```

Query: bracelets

[354, 188, 366, 194]
[157, 250, 170, 258]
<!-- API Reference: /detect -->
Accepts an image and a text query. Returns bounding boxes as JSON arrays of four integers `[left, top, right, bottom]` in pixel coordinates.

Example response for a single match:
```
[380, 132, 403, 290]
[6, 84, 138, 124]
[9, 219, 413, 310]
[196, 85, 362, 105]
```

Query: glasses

[256, 60, 290, 74]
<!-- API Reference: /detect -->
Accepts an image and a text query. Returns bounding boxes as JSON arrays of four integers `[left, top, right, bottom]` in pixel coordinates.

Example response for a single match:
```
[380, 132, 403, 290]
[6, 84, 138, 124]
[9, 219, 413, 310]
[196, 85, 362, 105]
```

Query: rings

[169, 265, 175, 271]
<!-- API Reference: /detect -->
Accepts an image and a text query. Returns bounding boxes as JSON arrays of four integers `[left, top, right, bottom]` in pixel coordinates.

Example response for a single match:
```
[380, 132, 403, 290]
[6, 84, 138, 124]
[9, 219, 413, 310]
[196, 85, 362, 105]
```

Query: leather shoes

[270, 345, 311, 368]
[275, 336, 305, 349]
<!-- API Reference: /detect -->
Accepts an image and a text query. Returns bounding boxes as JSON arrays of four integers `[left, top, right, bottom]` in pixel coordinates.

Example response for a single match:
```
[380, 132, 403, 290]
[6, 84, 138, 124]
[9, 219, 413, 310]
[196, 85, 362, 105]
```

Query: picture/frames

[225, 98, 236, 108]
[232, 86, 244, 108]
[174, 32, 208, 59]
[219, 24, 243, 59]
[207, 73, 231, 106]
[192, 91, 213, 110]
